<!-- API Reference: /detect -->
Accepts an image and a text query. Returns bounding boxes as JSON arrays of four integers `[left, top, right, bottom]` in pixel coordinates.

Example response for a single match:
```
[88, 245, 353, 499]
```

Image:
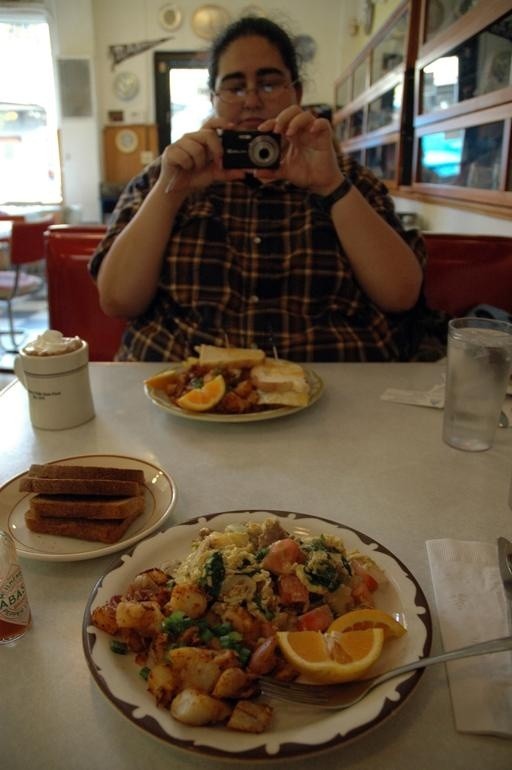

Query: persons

[88, 15, 427, 363]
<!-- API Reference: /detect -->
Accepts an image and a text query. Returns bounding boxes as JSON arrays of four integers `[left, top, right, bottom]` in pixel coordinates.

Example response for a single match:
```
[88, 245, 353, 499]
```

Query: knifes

[497, 535, 512, 632]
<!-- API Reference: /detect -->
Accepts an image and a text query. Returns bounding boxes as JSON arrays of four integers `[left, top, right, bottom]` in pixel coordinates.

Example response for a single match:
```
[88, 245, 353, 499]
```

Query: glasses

[209, 77, 301, 105]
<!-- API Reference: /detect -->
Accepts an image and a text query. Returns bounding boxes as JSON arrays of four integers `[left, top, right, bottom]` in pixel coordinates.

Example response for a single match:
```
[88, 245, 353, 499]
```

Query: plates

[2, 453, 177, 562]
[78, 505, 435, 763]
[137, 362, 325, 424]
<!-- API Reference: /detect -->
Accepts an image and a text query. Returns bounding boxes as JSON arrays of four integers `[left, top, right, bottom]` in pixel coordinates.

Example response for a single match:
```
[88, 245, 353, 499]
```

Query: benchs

[41, 224, 512, 363]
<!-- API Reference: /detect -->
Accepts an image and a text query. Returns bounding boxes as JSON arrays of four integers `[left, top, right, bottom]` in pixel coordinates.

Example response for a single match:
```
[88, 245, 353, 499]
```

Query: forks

[248, 633, 512, 716]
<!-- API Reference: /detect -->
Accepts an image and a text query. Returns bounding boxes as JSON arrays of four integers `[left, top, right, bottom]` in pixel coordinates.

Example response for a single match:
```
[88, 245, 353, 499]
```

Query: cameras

[221, 130, 280, 169]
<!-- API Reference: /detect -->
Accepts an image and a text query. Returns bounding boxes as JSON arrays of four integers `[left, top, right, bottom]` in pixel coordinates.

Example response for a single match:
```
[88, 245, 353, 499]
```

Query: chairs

[0, 215, 59, 344]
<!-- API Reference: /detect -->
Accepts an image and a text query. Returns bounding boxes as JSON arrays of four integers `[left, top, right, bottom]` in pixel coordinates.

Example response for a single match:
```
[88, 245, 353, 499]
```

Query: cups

[13, 335, 97, 436]
[433, 317, 507, 455]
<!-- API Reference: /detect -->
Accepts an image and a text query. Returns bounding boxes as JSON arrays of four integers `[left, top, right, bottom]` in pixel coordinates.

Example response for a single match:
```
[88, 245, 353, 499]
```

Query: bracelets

[323, 174, 352, 207]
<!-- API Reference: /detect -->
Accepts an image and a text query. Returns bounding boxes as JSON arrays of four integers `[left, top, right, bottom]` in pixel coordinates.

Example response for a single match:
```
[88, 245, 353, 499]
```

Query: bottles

[1, 528, 35, 649]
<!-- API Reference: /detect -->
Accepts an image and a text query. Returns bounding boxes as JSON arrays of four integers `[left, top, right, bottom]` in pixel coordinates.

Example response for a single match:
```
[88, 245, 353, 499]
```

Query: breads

[197, 343, 311, 409]
[18, 462, 146, 546]
